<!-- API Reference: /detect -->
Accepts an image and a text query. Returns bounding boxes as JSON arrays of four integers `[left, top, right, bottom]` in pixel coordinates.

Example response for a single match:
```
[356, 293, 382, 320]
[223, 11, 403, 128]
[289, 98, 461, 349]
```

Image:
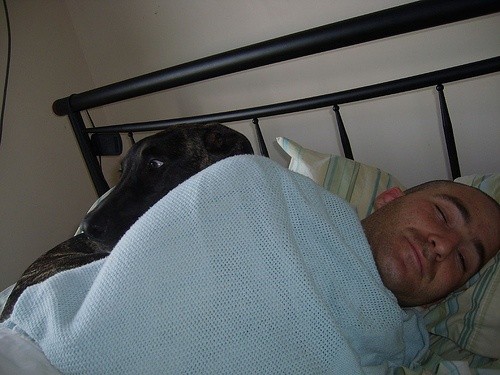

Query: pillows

[276, 137, 500, 358]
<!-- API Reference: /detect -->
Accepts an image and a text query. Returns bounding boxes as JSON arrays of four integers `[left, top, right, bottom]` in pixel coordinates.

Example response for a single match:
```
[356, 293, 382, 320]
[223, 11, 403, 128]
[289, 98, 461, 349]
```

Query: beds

[0, 0, 497, 375]
[0, 0, 500, 375]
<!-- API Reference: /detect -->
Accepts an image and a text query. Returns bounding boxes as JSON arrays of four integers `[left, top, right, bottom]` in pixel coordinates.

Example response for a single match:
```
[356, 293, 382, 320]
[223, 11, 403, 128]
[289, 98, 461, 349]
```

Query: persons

[0, 154, 500, 375]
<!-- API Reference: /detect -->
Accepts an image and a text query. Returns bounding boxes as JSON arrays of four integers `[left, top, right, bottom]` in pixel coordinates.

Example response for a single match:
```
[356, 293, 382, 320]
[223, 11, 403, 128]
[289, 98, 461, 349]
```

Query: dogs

[0, 122, 255, 323]
